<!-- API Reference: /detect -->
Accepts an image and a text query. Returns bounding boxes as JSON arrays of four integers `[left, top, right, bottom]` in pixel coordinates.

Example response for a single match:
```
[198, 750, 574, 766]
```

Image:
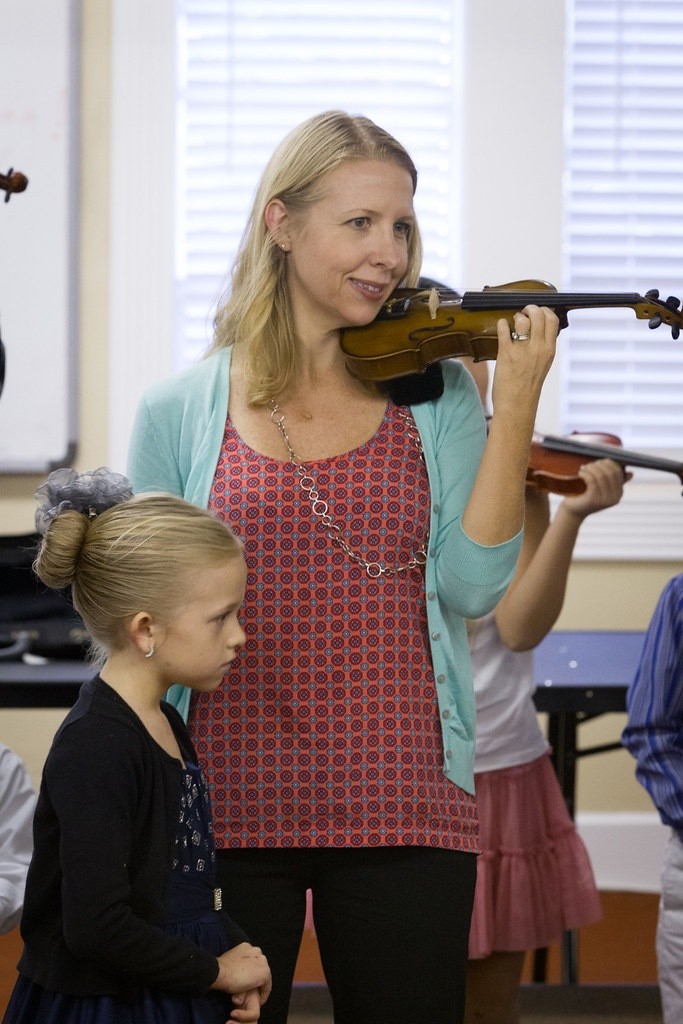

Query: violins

[483, 409, 683, 500]
[340, 280, 683, 379]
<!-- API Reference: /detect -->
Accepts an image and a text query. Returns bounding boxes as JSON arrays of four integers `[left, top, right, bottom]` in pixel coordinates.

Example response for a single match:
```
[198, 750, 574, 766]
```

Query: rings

[512, 332, 529, 341]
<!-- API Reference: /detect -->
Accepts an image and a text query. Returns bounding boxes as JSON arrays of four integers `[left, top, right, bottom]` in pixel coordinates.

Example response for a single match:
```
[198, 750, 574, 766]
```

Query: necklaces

[267, 395, 432, 578]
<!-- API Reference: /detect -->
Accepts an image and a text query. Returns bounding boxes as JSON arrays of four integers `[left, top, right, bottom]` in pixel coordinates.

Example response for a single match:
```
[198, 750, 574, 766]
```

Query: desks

[0, 627, 647, 987]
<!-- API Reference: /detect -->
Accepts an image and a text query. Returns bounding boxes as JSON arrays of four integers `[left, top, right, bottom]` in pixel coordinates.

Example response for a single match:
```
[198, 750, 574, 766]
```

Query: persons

[0, 742, 39, 936]
[621, 573, 683, 1024]
[3, 464, 273, 1024]
[452, 353, 634, 1024]
[123, 110, 560, 1024]
